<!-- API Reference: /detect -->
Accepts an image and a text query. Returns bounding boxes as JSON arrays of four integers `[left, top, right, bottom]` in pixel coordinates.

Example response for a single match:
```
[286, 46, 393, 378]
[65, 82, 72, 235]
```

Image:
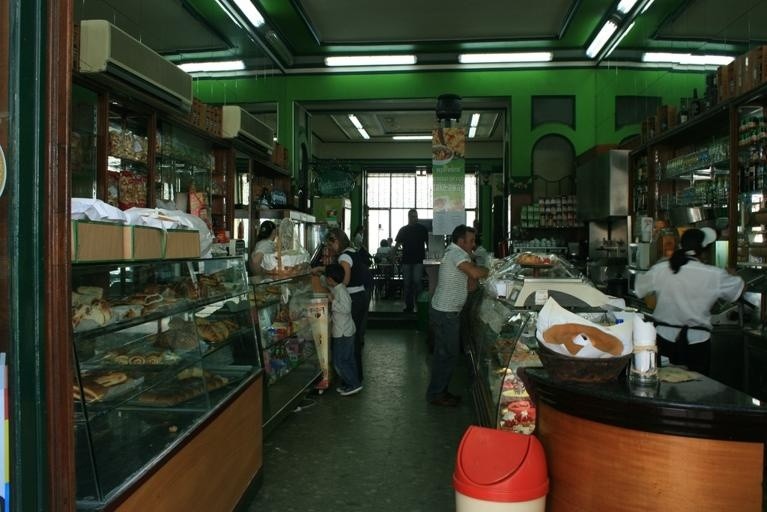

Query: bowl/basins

[432, 144, 455, 165]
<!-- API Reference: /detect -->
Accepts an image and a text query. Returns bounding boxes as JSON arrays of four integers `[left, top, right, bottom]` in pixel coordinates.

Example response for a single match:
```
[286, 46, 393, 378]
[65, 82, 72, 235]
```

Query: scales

[504, 265, 611, 310]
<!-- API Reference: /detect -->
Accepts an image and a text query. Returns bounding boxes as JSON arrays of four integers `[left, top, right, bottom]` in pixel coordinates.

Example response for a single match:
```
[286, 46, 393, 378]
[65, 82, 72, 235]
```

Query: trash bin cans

[415, 291, 431, 335]
[452, 426, 550, 511]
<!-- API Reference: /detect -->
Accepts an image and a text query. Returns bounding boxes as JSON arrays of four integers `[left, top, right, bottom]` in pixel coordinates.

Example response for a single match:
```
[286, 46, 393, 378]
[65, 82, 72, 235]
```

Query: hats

[679, 226, 717, 255]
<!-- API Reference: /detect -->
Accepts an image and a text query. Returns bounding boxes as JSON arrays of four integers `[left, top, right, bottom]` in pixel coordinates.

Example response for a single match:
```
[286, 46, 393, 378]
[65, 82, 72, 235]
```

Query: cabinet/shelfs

[72, 73, 335, 511]
[459, 41, 767, 511]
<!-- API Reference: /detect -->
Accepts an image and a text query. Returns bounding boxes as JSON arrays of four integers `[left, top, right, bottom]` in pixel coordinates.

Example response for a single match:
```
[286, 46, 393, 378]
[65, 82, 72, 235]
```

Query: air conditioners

[80, 20, 192, 119]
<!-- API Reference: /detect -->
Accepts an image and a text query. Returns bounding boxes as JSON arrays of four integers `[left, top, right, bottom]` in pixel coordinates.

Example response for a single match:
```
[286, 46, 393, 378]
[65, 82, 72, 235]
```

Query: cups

[627, 349, 660, 399]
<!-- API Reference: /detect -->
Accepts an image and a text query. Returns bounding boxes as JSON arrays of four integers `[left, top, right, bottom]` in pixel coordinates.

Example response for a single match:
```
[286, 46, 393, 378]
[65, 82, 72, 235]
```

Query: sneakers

[403, 306, 414, 313]
[336, 384, 363, 396]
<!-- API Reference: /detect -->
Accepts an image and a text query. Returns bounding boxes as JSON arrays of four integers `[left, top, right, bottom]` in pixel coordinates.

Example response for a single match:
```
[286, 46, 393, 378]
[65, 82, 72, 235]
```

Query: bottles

[690, 87, 701, 115]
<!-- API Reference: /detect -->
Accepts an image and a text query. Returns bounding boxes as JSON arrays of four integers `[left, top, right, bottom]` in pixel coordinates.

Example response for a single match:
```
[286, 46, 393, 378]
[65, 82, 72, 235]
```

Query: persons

[248, 220, 276, 272]
[425, 224, 489, 408]
[635, 228, 748, 382]
[389, 209, 428, 311]
[353, 225, 364, 245]
[375, 239, 391, 254]
[309, 227, 371, 384]
[315, 262, 364, 396]
[387, 238, 393, 247]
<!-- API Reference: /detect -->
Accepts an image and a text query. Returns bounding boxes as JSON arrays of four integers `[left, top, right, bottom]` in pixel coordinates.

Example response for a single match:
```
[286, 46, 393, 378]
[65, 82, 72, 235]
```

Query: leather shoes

[429, 391, 463, 408]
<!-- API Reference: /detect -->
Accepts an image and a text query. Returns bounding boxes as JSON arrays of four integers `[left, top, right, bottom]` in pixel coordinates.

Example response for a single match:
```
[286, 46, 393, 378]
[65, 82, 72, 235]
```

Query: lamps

[392, 135, 434, 140]
[177, 0, 729, 73]
[468, 113, 481, 139]
[349, 112, 371, 140]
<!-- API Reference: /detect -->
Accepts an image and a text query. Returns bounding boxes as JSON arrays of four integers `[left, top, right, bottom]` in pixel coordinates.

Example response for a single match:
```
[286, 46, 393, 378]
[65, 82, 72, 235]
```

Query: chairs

[371, 253, 407, 304]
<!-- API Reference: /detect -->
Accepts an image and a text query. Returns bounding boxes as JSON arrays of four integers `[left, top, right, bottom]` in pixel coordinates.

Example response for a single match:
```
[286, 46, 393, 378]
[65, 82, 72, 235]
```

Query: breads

[73, 277, 239, 405]
[517, 255, 542, 265]
